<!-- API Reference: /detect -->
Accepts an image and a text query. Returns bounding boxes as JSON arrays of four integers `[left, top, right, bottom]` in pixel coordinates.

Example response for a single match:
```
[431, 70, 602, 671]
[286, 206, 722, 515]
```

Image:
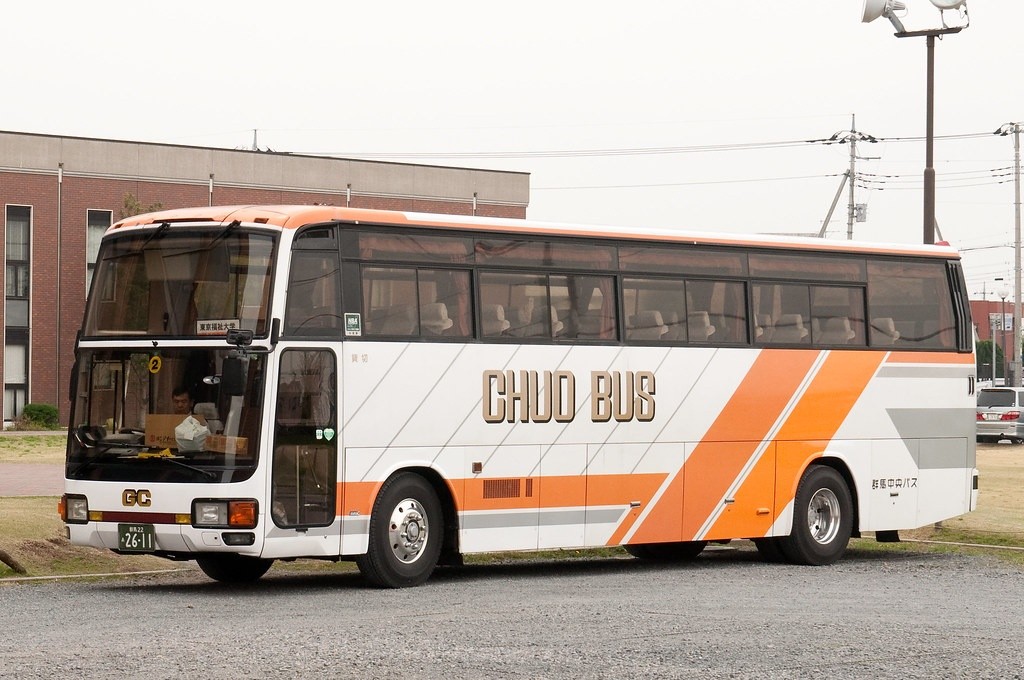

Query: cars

[977, 387, 1024, 444]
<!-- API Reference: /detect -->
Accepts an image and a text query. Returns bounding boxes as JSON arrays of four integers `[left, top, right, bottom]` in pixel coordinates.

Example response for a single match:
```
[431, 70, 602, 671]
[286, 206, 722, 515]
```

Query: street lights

[999, 291, 1010, 387]
[861, 0, 970, 245]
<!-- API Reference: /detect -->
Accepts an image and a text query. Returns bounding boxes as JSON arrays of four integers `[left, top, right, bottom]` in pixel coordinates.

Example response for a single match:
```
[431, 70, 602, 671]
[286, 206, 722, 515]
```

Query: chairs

[238, 296, 905, 353]
[194, 401, 225, 437]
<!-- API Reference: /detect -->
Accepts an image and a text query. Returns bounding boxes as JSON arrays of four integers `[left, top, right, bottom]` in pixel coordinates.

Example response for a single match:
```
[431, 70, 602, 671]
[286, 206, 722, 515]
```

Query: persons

[170, 386, 195, 415]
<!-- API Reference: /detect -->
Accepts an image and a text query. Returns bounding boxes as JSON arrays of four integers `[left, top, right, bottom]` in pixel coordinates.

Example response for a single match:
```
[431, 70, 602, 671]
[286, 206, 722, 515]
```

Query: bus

[55, 204, 977, 589]
